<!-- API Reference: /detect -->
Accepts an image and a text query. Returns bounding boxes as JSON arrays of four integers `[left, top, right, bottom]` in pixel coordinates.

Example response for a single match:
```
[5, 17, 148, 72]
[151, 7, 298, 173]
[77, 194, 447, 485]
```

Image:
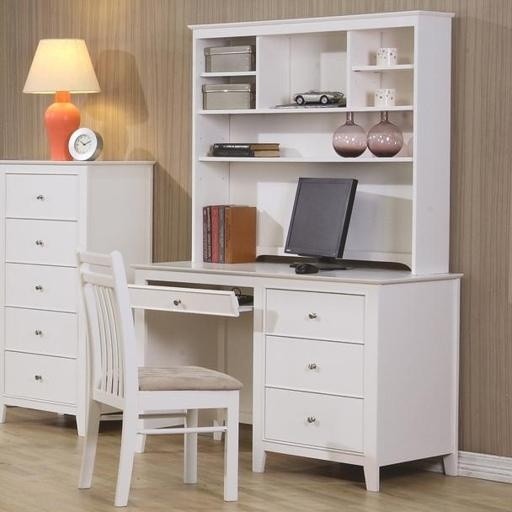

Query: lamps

[21, 37, 102, 160]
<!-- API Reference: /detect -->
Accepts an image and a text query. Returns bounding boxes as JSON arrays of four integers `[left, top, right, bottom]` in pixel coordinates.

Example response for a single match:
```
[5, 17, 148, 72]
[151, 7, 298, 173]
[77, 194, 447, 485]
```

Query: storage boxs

[201, 43, 255, 110]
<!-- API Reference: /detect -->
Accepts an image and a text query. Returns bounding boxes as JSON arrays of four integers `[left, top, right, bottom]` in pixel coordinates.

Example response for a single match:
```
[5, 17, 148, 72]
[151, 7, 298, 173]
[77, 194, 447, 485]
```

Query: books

[213, 142, 281, 157]
[203, 204, 257, 264]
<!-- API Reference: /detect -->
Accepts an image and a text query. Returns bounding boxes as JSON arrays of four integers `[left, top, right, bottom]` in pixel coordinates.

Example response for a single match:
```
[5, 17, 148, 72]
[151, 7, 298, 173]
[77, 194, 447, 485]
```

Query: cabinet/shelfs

[186, 11, 458, 275]
[0, 160, 155, 438]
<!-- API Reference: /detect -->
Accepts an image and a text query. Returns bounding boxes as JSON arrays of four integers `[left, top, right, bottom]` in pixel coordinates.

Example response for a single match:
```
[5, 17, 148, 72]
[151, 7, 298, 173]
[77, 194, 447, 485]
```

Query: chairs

[74, 248, 243, 506]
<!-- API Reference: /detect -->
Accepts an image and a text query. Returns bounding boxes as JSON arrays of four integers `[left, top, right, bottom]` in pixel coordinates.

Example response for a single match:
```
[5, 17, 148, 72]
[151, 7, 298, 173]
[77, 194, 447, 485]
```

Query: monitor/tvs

[284, 177, 358, 270]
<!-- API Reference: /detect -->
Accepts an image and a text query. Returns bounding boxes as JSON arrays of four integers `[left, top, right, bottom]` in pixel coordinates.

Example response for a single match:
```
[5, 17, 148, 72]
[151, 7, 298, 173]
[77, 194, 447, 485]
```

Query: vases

[332, 110, 405, 157]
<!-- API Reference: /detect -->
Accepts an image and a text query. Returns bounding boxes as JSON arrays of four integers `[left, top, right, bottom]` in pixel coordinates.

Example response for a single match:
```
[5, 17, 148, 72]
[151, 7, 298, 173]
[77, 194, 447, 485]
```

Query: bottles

[332, 112, 404, 158]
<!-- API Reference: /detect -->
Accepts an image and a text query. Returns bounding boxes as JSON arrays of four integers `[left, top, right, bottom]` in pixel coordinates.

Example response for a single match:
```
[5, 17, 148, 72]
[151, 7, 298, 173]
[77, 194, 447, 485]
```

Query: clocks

[68, 127, 104, 161]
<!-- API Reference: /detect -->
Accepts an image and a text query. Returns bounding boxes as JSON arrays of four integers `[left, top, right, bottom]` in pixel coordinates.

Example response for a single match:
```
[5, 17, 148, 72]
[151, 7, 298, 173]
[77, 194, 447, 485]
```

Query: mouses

[295, 264, 318, 273]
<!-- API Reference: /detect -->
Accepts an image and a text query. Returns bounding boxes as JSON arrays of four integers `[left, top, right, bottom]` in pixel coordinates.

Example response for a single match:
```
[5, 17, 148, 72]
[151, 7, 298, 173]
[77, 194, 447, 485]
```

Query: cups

[373, 89, 396, 105]
[377, 49, 398, 66]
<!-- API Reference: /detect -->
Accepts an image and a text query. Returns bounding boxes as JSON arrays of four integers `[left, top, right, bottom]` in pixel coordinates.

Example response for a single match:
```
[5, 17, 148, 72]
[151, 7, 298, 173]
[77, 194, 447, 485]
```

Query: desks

[125, 259, 463, 492]
